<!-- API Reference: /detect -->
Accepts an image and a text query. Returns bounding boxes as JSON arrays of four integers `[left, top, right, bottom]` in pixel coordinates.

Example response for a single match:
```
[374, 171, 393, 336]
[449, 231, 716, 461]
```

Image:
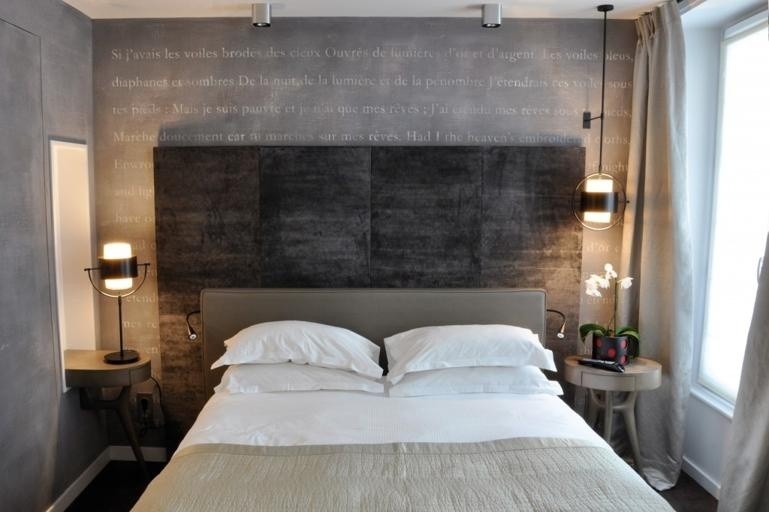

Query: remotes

[578, 357, 625, 373]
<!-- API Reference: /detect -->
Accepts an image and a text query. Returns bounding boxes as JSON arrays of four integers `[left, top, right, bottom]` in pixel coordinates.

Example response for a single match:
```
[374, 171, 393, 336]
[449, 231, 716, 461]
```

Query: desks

[63, 350, 152, 483]
[565, 353, 663, 482]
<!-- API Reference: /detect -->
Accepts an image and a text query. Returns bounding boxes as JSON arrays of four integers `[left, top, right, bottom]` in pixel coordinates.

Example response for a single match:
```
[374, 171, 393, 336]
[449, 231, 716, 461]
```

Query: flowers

[579, 263, 641, 359]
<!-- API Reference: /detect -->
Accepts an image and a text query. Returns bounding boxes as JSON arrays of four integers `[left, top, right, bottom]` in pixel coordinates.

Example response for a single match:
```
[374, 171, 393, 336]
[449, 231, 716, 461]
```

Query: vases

[592, 332, 630, 366]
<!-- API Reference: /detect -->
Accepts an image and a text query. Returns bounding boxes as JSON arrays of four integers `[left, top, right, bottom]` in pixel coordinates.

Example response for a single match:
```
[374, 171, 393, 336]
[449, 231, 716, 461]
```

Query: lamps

[252, 4, 271, 27]
[571, 5, 630, 232]
[547, 309, 566, 338]
[482, 5, 500, 28]
[83, 242, 150, 363]
[185, 310, 201, 341]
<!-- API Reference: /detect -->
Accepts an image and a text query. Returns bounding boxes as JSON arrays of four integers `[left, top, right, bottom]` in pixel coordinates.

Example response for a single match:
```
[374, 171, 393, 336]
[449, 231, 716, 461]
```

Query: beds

[126, 286, 677, 512]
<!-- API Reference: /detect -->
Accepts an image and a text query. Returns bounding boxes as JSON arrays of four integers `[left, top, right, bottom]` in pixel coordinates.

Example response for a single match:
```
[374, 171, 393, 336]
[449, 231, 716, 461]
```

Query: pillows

[211, 321, 564, 393]
